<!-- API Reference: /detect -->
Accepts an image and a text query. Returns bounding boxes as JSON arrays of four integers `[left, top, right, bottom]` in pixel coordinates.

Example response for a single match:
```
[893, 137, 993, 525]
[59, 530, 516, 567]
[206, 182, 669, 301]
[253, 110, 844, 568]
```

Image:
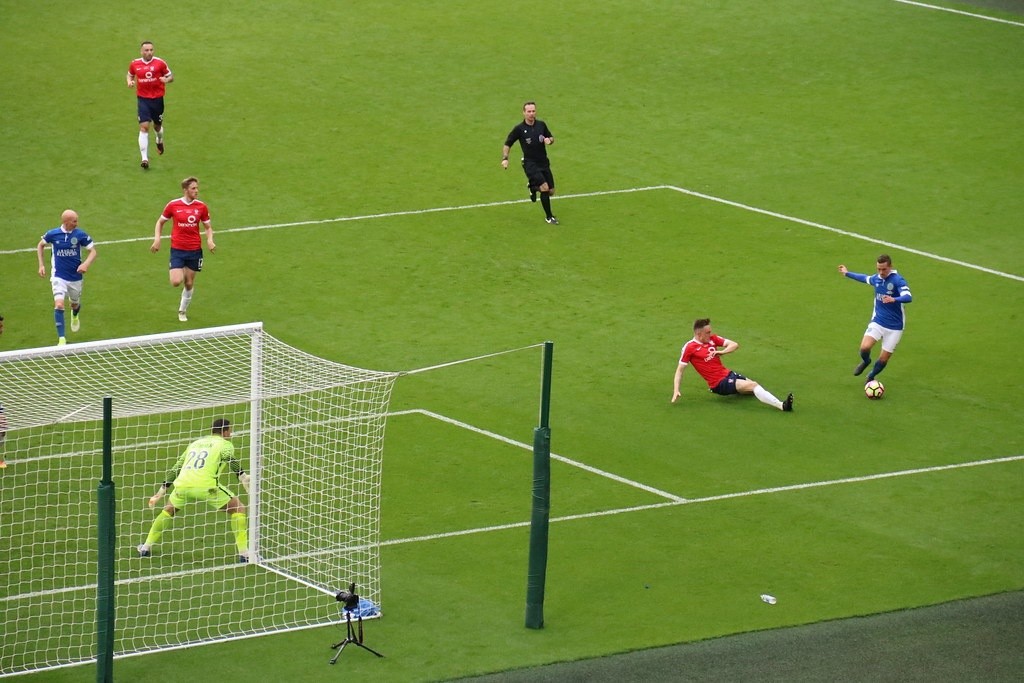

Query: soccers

[865, 380, 885, 400]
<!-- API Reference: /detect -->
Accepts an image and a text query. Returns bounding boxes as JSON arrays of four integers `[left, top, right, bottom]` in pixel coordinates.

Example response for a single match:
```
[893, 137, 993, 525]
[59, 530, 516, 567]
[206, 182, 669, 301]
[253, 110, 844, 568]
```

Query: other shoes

[864, 375, 875, 385]
[0, 460, 7, 468]
[854, 358, 871, 376]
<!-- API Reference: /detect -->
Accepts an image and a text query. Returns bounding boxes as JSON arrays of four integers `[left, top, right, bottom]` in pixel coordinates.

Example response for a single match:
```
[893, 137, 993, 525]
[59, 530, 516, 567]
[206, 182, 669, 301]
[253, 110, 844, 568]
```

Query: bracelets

[503, 156, 508, 160]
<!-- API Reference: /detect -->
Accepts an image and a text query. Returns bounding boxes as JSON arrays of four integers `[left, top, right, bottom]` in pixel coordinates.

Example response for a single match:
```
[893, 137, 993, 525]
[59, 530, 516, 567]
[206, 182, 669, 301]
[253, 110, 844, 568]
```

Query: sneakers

[545, 216, 559, 225]
[527, 183, 536, 203]
[71, 310, 80, 333]
[156, 138, 164, 155]
[178, 308, 188, 322]
[141, 160, 149, 169]
[57, 337, 66, 346]
[240, 552, 264, 563]
[783, 393, 794, 411]
[137, 544, 149, 556]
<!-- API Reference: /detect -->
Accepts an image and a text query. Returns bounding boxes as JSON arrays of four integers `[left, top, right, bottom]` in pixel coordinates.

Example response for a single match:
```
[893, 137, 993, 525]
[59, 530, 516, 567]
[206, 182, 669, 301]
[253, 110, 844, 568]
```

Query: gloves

[149, 486, 166, 507]
[238, 472, 250, 493]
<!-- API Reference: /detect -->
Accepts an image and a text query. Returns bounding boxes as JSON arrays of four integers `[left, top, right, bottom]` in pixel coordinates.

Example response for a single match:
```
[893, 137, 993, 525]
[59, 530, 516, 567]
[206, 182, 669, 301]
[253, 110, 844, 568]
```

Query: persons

[38, 209, 96, 346]
[838, 253, 912, 384]
[127, 41, 173, 169]
[137, 418, 250, 562]
[501, 101, 559, 224]
[671, 319, 793, 411]
[150, 177, 216, 321]
